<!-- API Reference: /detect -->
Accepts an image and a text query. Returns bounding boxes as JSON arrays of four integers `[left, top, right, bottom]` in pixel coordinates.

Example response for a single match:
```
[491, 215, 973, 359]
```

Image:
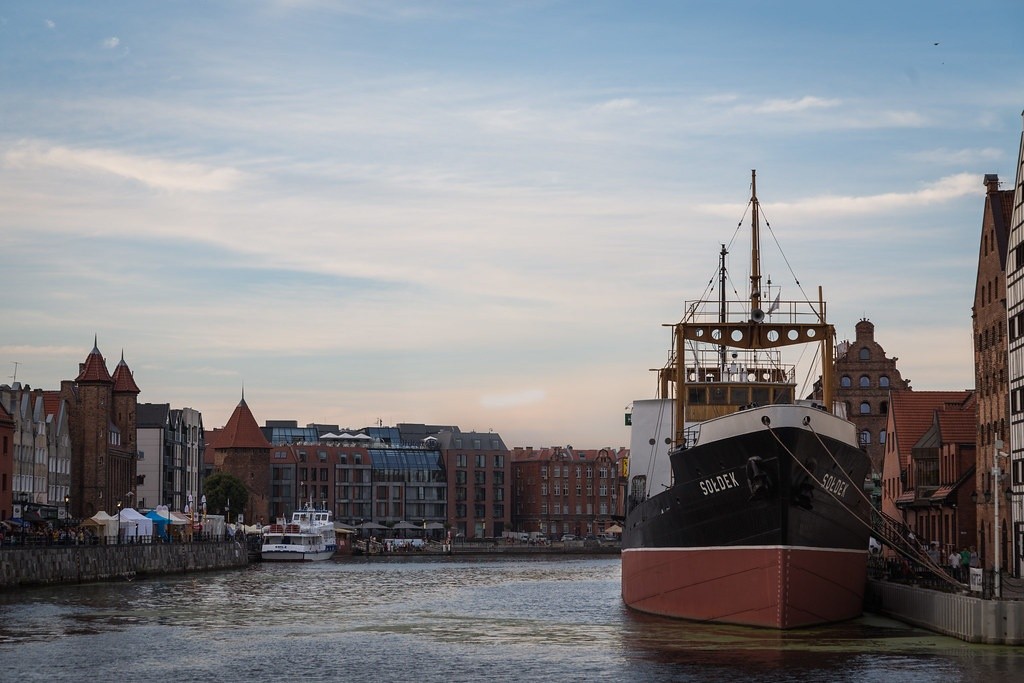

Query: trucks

[502, 530, 617, 544]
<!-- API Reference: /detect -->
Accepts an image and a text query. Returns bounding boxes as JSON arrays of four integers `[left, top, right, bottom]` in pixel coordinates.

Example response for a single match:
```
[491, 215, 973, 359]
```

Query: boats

[618, 169, 877, 630]
[262, 494, 339, 563]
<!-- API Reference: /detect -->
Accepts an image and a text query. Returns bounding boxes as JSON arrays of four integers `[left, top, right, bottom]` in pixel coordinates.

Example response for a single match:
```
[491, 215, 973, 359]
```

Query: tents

[84, 509, 193, 545]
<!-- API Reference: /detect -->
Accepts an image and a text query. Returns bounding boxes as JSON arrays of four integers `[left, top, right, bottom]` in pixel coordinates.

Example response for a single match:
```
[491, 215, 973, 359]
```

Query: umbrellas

[605, 525, 622, 538]
[331, 521, 457, 539]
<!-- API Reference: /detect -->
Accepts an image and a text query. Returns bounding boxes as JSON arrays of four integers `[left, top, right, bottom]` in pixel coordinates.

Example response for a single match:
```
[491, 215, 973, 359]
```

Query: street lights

[20, 492, 28, 546]
[224, 504, 229, 542]
[260, 516, 264, 550]
[167, 501, 172, 542]
[115, 497, 123, 546]
[990, 432, 1009, 599]
[64, 494, 72, 545]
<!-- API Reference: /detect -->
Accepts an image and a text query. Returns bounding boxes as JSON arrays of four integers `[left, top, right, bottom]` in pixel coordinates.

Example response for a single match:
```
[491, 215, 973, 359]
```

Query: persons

[927, 543, 979, 587]
[384, 540, 430, 552]
[172, 527, 178, 542]
[179, 528, 185, 542]
[0, 522, 92, 546]
